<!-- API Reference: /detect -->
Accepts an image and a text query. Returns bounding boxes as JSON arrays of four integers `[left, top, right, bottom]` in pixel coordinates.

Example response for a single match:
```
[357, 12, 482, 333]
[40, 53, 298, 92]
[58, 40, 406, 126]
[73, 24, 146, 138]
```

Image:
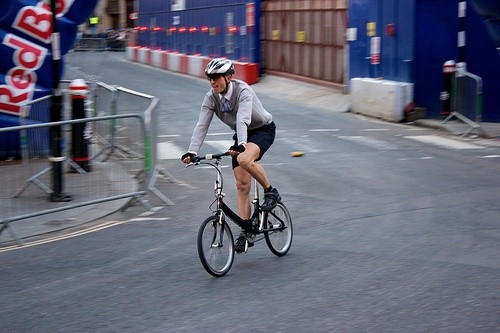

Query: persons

[182, 58, 282, 250]
[77, 29, 131, 49]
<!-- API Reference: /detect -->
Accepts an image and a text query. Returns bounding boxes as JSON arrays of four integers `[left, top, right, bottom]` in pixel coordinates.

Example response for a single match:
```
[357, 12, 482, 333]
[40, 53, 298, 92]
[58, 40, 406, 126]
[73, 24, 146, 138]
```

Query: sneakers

[233, 229, 254, 252]
[261, 188, 281, 211]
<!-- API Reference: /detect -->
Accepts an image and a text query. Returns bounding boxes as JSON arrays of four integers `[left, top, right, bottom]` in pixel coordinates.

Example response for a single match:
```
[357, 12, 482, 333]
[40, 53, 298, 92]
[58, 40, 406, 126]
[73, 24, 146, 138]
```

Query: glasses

[207, 75, 228, 81]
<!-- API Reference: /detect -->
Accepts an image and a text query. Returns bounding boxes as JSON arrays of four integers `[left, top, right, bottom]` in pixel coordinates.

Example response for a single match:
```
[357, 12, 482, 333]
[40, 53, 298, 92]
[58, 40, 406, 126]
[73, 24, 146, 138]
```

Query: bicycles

[186, 151, 293, 277]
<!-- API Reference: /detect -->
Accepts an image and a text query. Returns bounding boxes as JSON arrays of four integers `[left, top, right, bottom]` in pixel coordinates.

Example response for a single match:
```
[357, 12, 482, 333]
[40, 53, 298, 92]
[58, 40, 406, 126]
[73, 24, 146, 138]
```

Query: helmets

[204, 58, 235, 76]
